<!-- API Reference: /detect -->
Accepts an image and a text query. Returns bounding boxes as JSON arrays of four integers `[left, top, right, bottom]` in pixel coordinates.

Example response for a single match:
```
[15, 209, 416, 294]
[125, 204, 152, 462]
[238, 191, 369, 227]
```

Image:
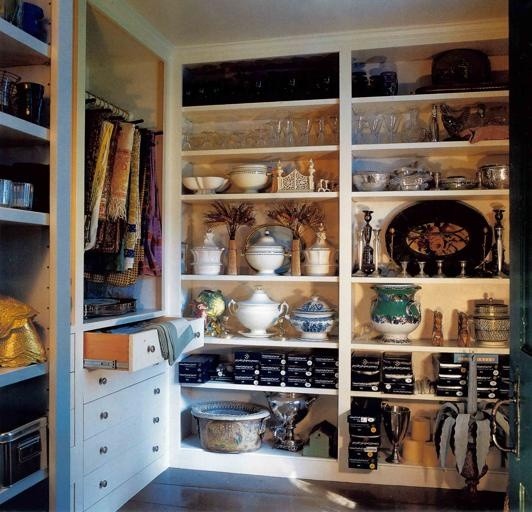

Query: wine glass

[179, 111, 340, 151]
[399, 259, 471, 277]
[352, 105, 425, 145]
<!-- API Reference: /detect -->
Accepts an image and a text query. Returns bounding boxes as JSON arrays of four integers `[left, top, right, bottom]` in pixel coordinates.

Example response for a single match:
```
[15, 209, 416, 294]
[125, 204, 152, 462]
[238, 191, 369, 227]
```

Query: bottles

[429, 103, 439, 142]
[457, 311, 471, 348]
[472, 297, 509, 343]
[190, 231, 226, 276]
[288, 295, 340, 343]
[431, 311, 444, 346]
[243, 231, 292, 275]
[303, 224, 337, 276]
[228, 288, 290, 339]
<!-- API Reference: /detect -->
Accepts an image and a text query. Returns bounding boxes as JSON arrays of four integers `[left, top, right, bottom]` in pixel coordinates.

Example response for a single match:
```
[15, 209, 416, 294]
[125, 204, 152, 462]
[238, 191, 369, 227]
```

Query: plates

[385, 199, 492, 277]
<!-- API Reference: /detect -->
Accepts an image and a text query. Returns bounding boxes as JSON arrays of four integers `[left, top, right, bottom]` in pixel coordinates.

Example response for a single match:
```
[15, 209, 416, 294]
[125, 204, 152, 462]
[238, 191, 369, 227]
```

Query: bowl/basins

[352, 163, 509, 192]
[0, 0, 48, 42]
[182, 177, 232, 195]
[190, 401, 271, 453]
[428, 50, 492, 87]
[225, 161, 273, 194]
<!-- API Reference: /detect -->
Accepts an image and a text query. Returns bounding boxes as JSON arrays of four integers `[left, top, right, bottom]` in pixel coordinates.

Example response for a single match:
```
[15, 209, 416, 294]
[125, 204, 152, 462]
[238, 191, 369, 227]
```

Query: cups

[367, 284, 422, 344]
[352, 70, 399, 96]
[0, 179, 33, 209]
[0, 70, 43, 124]
